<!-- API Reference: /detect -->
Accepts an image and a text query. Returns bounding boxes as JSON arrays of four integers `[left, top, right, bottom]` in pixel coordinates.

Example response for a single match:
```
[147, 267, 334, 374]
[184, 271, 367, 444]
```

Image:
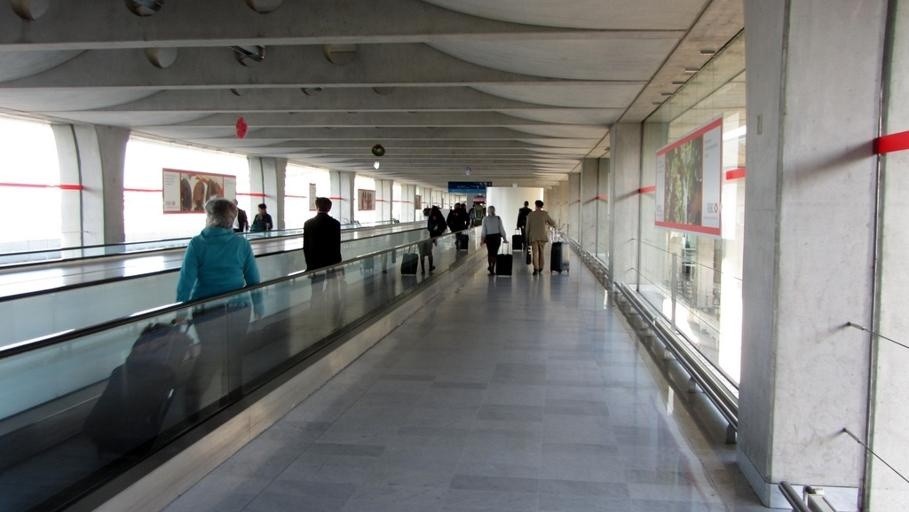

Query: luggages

[512, 228, 522, 250]
[401, 244, 418, 274]
[551, 227, 569, 274]
[83, 318, 192, 459]
[460, 234, 468, 249]
[496, 241, 512, 275]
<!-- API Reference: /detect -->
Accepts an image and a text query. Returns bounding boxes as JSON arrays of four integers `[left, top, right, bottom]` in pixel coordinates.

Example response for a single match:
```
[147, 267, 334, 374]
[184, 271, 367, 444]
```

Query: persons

[232, 200, 248, 233]
[302, 198, 342, 310]
[250, 204, 272, 231]
[428, 202, 556, 276]
[418, 239, 436, 274]
[176, 199, 265, 416]
[181, 176, 216, 210]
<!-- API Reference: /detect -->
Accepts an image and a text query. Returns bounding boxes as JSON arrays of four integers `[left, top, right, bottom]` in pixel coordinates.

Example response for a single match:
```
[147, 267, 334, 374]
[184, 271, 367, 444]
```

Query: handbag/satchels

[526, 247, 531, 264]
